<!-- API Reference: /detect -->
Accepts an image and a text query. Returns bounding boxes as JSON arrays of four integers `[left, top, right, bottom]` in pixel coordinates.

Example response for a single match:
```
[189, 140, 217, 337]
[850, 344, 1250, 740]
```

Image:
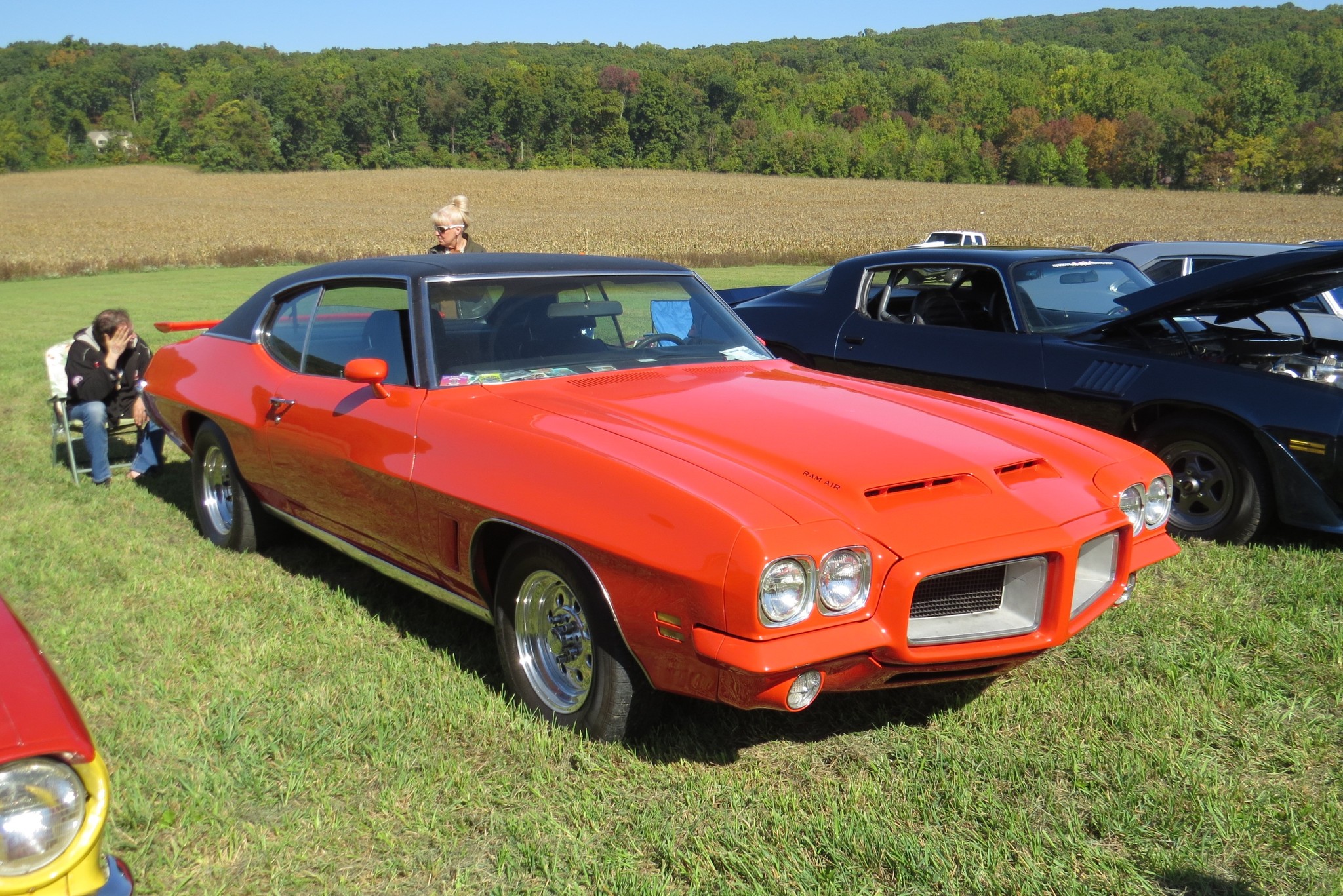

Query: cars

[139, 246, 1177, 731]
[677, 242, 1343, 548]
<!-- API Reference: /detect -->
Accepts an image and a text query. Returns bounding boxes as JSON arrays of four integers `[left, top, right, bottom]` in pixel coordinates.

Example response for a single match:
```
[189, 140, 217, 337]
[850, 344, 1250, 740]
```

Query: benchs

[281, 326, 510, 377]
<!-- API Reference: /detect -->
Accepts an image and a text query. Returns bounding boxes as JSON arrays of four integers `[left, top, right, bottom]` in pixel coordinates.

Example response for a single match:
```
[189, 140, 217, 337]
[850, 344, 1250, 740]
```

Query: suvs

[1102, 234, 1342, 346]
[910, 231, 983, 252]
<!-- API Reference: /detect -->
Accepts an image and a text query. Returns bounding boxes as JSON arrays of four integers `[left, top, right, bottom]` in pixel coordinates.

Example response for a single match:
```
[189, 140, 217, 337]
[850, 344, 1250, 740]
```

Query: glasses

[433, 225, 465, 233]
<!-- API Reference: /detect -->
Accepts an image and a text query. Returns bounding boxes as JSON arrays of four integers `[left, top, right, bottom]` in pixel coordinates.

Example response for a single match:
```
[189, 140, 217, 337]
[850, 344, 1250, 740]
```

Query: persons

[63, 307, 170, 485]
[425, 195, 496, 319]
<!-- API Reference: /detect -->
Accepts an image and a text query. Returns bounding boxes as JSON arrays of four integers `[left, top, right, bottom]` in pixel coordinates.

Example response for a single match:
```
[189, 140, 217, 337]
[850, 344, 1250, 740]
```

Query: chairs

[1224, 337, 1305, 368]
[991, 285, 1048, 329]
[359, 307, 449, 386]
[45, 339, 146, 485]
[516, 304, 608, 359]
[908, 288, 970, 328]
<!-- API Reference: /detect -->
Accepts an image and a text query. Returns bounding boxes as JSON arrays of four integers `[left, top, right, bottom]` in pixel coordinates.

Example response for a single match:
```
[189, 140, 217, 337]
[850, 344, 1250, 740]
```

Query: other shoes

[95, 478, 112, 490]
[125, 471, 151, 482]
